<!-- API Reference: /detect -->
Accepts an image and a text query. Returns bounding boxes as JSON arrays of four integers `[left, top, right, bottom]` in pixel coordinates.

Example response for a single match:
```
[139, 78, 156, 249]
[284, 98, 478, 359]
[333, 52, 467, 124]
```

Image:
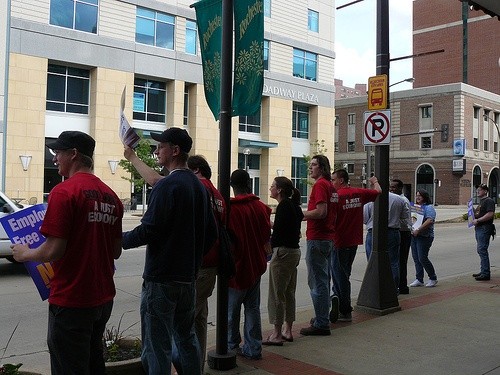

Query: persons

[330, 168, 382, 322]
[389, 191, 409, 297]
[408, 189, 438, 287]
[472, 183, 494, 281]
[121, 146, 227, 375]
[207, 169, 272, 359]
[361, 199, 374, 262]
[389, 178, 412, 294]
[263, 176, 304, 346]
[300, 155, 340, 336]
[121, 127, 218, 375]
[9, 131, 124, 375]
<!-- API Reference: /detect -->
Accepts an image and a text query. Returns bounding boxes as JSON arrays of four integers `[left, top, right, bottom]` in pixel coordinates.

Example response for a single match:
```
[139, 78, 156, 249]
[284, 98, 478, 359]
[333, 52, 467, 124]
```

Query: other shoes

[329, 295, 339, 323]
[235, 347, 261, 360]
[475, 275, 490, 280]
[337, 315, 352, 321]
[310, 317, 316, 327]
[300, 325, 332, 336]
[472, 272, 482, 277]
[398, 289, 409, 294]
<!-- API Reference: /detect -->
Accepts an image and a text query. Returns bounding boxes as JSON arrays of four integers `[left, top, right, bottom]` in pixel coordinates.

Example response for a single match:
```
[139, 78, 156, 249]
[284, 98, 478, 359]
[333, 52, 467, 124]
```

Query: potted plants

[104, 310, 144, 375]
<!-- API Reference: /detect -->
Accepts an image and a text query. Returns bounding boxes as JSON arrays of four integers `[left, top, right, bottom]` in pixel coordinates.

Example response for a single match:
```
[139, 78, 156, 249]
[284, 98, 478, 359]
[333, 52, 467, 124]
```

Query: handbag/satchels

[489, 224, 496, 240]
[217, 220, 236, 276]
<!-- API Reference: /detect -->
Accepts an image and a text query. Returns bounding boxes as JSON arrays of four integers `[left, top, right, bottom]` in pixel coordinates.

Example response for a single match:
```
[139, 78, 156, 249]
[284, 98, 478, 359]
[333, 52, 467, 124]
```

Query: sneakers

[410, 279, 424, 286]
[426, 279, 439, 287]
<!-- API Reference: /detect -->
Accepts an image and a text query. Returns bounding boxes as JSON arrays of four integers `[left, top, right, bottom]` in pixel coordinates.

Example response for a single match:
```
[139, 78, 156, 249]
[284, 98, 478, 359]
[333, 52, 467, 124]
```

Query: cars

[0, 191, 38, 264]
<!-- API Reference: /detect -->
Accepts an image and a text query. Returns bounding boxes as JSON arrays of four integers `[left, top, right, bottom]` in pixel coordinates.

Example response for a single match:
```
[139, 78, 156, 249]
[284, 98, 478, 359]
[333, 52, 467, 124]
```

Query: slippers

[280, 333, 294, 342]
[262, 334, 283, 346]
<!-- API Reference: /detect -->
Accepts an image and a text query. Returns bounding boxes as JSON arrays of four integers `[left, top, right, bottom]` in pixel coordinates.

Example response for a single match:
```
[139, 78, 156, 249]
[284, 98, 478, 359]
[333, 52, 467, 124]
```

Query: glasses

[156, 144, 177, 151]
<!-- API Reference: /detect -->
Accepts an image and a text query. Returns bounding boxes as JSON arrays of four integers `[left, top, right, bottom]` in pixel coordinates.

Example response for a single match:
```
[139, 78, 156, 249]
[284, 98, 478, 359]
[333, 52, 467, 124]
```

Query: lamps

[109, 160, 119, 174]
[19, 155, 32, 171]
[277, 169, 284, 176]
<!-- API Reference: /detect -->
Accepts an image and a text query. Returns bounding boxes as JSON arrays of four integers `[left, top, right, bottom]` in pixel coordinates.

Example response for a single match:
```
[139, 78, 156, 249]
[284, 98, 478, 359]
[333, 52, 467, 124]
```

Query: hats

[150, 127, 193, 153]
[45, 131, 95, 158]
[475, 183, 489, 192]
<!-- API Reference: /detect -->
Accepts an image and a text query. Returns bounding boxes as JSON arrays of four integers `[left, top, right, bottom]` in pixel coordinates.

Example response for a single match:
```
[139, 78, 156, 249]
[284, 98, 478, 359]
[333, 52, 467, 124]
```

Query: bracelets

[476, 218, 480, 223]
[373, 180, 378, 184]
